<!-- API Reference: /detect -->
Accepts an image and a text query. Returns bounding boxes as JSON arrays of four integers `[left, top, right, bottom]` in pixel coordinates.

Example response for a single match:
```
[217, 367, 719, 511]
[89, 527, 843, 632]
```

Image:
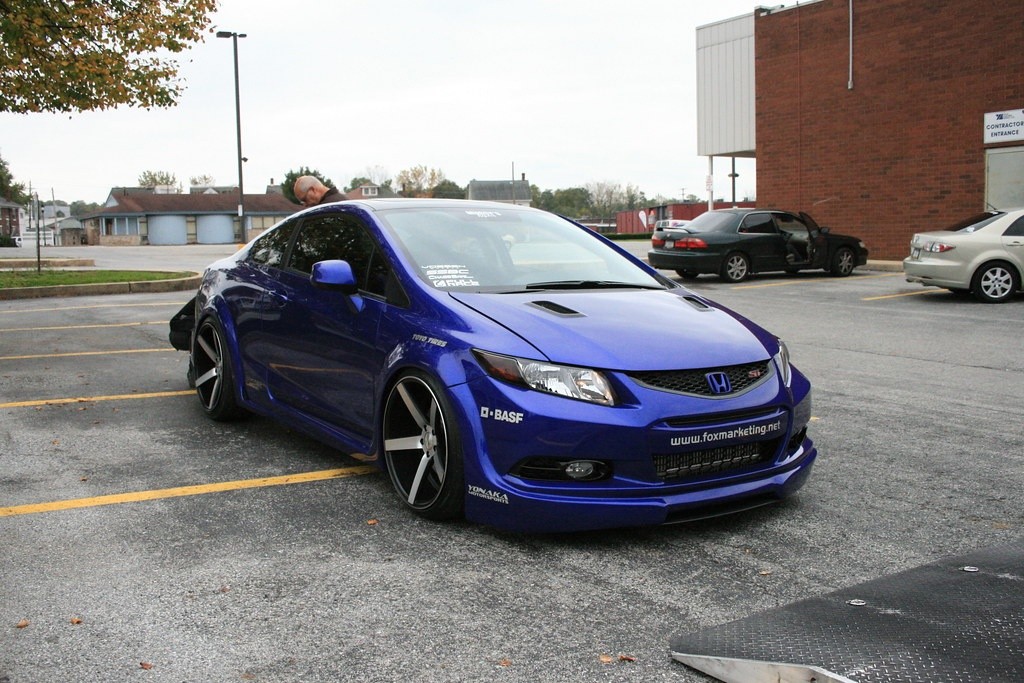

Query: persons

[292, 174, 349, 207]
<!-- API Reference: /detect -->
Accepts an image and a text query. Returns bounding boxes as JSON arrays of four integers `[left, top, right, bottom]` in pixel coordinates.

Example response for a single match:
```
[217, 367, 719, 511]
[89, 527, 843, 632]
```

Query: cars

[903, 207, 1024, 302]
[647, 207, 868, 284]
[188, 199, 816, 537]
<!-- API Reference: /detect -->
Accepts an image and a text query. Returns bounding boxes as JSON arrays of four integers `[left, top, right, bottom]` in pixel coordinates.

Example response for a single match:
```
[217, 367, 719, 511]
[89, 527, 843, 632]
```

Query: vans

[11, 237, 21, 247]
[652, 220, 689, 236]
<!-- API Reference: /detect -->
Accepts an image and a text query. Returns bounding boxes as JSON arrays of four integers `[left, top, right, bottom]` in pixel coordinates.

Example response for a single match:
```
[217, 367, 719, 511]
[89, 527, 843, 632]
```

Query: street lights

[217, 31, 248, 245]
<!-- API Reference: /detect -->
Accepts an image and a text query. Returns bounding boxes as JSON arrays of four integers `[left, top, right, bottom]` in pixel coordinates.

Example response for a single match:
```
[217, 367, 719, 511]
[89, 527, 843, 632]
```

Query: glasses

[300, 188, 310, 204]
